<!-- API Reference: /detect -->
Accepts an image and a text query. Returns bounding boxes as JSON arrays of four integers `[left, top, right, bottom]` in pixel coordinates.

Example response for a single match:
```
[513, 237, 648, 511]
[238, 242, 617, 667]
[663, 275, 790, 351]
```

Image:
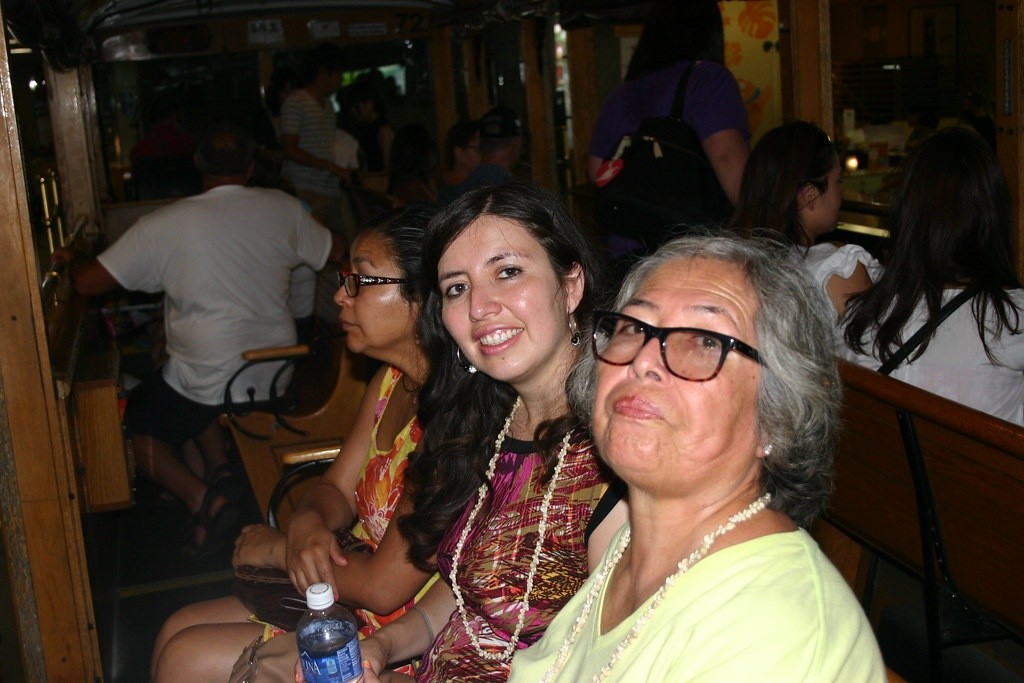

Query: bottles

[295, 582, 363, 682]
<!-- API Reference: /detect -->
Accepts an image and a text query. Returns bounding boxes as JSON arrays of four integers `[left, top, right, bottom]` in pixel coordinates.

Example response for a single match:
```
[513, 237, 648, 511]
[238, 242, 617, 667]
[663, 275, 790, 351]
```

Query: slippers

[211, 464, 241, 502]
[183, 487, 241, 562]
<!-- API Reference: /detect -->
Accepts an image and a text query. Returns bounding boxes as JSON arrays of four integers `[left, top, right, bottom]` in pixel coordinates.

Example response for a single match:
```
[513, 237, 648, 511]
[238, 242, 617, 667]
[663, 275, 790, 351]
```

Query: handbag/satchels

[592, 58, 735, 243]
[230, 630, 417, 683]
[232, 528, 375, 629]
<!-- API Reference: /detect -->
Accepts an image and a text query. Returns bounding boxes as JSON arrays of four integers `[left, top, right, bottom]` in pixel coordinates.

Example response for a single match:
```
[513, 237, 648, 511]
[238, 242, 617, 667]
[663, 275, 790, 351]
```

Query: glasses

[336, 272, 409, 297]
[592, 309, 766, 382]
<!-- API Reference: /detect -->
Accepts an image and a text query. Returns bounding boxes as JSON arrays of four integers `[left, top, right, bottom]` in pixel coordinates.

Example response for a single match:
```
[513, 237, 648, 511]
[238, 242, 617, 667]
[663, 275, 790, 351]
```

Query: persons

[583, 0, 756, 295]
[122, 37, 572, 241]
[503, 234, 890, 683]
[294, 178, 637, 681]
[74, 120, 348, 570]
[834, 120, 1024, 428]
[734, 120, 894, 381]
[146, 209, 495, 682]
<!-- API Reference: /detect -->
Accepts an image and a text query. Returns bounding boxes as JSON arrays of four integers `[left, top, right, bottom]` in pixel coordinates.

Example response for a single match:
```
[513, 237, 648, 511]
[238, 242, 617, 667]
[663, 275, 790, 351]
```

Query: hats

[479, 105, 533, 138]
[339, 76, 388, 112]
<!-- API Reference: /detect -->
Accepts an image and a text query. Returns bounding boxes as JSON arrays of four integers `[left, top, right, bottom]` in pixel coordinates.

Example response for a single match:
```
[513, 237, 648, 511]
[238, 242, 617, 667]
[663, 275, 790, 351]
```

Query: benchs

[221, 273, 1024, 683]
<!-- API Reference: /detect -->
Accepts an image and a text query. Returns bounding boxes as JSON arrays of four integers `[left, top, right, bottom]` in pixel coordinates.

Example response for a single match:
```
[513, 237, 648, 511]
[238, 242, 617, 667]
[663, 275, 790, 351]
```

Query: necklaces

[447, 390, 581, 660]
[541, 494, 773, 683]
